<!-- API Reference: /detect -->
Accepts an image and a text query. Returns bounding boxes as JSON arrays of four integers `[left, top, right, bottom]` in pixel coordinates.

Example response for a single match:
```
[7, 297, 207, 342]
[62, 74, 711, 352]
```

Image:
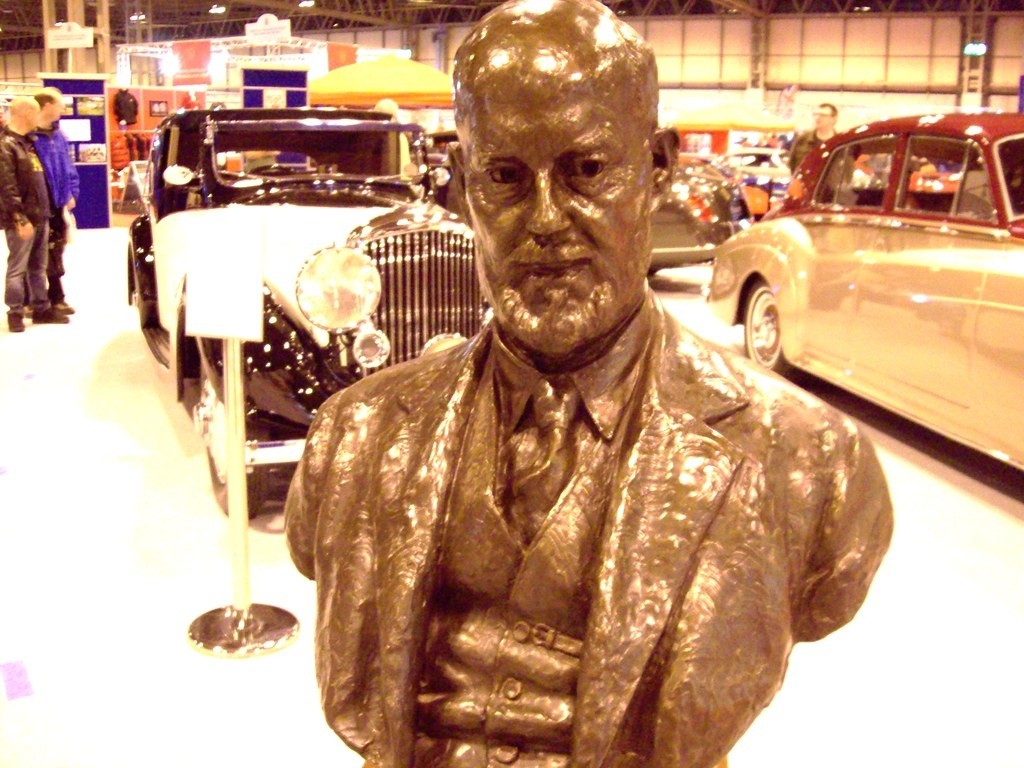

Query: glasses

[813, 112, 834, 119]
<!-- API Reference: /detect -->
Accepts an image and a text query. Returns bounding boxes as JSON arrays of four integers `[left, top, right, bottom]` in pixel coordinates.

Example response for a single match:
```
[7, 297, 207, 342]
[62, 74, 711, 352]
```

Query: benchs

[823, 188, 973, 213]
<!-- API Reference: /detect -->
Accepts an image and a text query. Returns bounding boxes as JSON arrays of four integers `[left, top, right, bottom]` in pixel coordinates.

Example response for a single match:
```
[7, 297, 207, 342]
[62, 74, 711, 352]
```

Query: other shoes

[7, 310, 25, 332]
[52, 302, 75, 314]
[24, 307, 34, 318]
[32, 309, 69, 324]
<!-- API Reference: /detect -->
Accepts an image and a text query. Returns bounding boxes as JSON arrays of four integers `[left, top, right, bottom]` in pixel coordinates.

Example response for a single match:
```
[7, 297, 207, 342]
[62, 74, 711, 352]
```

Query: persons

[736, 102, 951, 204]
[0, 87, 81, 332]
[283, 0, 893, 768]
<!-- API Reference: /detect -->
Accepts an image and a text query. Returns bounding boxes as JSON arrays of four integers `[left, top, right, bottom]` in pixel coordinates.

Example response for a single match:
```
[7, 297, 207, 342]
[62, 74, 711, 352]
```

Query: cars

[650, 151, 756, 276]
[708, 112, 1024, 474]
[126, 107, 475, 545]
[708, 146, 794, 188]
